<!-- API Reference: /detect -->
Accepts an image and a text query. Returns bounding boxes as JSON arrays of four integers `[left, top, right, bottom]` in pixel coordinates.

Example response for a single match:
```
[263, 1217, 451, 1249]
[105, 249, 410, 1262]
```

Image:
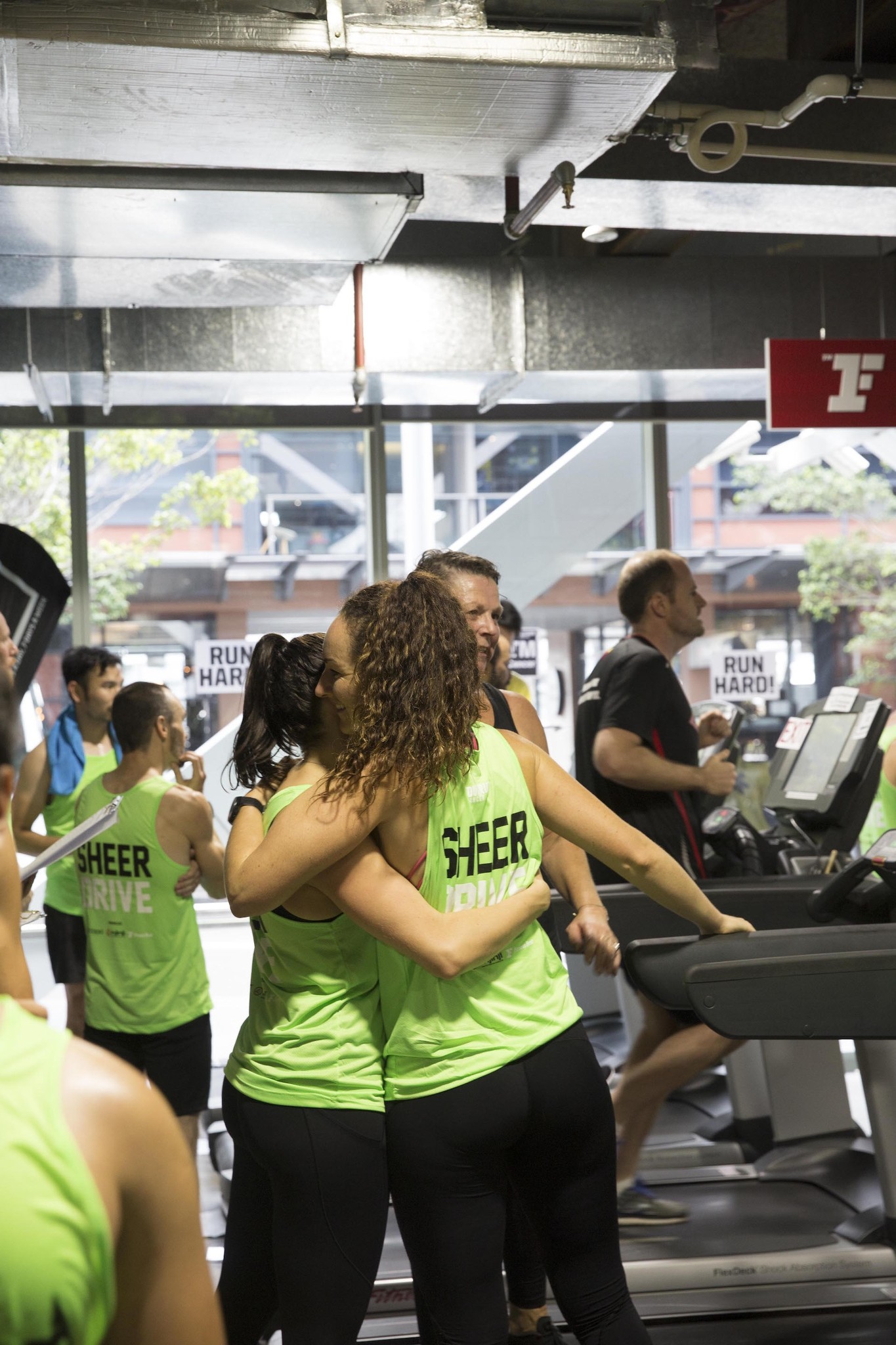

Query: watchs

[227, 796, 263, 823]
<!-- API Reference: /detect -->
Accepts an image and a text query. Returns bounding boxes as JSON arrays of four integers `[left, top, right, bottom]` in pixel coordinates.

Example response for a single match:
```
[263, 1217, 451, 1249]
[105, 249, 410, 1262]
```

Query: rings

[613, 942, 620, 950]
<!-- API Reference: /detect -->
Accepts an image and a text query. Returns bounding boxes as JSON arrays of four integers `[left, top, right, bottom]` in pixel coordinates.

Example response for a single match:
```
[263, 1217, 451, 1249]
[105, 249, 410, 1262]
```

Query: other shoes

[509, 1316, 568, 1345]
[613, 1187, 690, 1225]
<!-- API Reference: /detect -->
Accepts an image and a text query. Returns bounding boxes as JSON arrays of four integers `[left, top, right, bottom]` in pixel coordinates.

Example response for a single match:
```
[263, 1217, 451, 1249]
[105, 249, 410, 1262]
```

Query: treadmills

[202, 673, 896, 1218]
[660, 703, 746, 767]
[289, 826, 896, 1318]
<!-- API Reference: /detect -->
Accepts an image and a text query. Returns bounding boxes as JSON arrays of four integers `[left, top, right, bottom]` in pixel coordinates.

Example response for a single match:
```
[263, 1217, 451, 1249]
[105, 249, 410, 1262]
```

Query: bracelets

[573, 904, 609, 920]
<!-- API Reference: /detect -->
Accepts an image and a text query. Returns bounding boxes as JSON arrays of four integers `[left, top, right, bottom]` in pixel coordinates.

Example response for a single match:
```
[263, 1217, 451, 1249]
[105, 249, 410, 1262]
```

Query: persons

[493, 600, 530, 699]
[0, 993, 228, 1345]
[0, 613, 35, 1002]
[12, 645, 124, 1038]
[74, 681, 225, 1153]
[417, 549, 621, 974]
[219, 633, 551, 1345]
[230, 570, 758, 1345]
[575, 549, 737, 1225]
